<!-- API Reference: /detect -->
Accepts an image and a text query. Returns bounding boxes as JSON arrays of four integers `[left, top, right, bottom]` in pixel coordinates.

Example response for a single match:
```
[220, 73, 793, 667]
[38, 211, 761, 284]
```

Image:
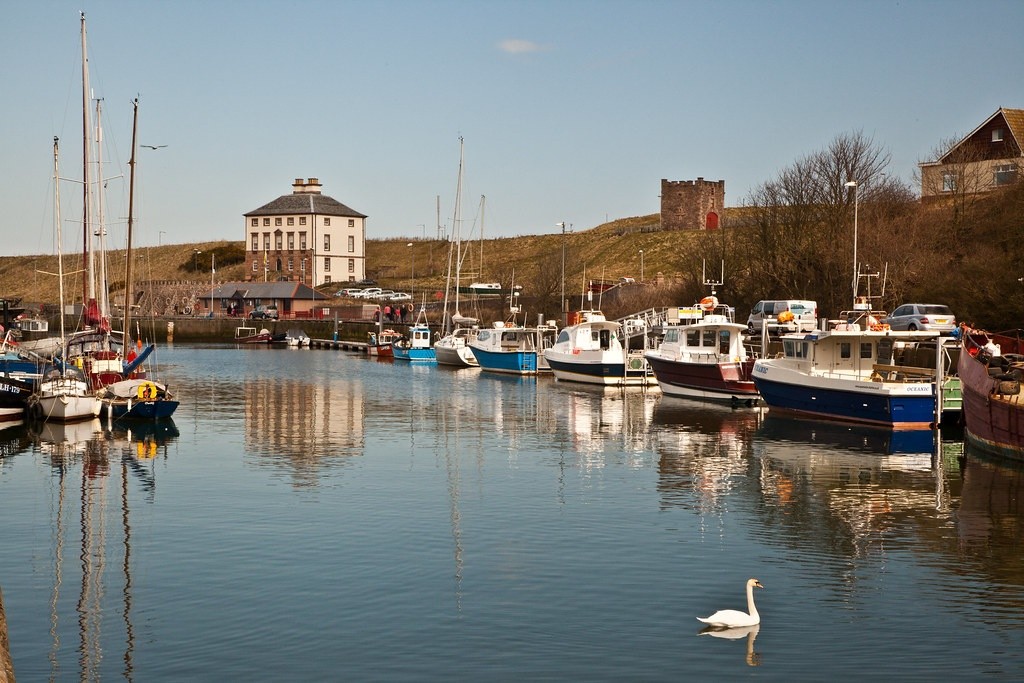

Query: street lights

[638, 249, 644, 281]
[555, 222, 566, 313]
[309, 248, 315, 320]
[194, 248, 202, 317]
[406, 242, 414, 323]
[159, 230, 167, 246]
[843, 180, 858, 306]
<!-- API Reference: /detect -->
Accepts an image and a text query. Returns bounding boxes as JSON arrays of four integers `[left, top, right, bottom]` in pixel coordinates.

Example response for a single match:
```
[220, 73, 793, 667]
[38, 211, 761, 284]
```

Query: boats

[233, 317, 272, 344]
[466, 259, 967, 429]
[366, 327, 403, 357]
[951, 320, 1024, 463]
[390, 290, 437, 362]
[0, 10, 181, 441]
[285, 328, 310, 349]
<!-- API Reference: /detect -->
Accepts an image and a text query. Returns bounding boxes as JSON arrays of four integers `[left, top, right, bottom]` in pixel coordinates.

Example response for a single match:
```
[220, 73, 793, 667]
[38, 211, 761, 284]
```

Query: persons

[384, 304, 407, 324]
[227, 305, 238, 316]
[949, 322, 988, 337]
[373, 307, 381, 322]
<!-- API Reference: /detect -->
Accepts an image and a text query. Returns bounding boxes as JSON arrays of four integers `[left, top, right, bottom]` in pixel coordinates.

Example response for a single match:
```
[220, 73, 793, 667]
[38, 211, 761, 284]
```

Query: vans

[746, 299, 818, 336]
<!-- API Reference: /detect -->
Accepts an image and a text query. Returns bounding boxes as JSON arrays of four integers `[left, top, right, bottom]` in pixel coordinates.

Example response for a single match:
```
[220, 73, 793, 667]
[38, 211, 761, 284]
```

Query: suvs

[248, 305, 280, 321]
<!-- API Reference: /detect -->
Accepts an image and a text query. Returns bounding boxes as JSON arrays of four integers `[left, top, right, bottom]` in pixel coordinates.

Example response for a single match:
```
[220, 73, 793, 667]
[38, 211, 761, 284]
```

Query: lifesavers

[138, 381, 157, 399]
[700, 296, 718, 311]
[408, 303, 413, 312]
[137, 441, 157, 459]
[77, 358, 82, 368]
[871, 324, 890, 331]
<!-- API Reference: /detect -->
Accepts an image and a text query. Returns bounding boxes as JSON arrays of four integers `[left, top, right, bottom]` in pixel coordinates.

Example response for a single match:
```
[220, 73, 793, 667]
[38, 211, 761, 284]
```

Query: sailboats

[432, 135, 488, 367]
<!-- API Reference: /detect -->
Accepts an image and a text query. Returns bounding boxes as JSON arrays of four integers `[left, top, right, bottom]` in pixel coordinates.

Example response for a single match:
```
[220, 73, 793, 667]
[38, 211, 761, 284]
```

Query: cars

[878, 302, 957, 342]
[332, 288, 414, 302]
[617, 276, 635, 285]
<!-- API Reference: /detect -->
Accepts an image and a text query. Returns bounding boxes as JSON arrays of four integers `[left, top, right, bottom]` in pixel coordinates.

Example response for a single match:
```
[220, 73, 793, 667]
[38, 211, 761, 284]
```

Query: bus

[587, 278, 622, 294]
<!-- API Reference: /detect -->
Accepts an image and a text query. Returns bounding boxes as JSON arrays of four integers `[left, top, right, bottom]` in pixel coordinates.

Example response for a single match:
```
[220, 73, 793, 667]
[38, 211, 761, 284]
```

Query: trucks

[469, 282, 502, 297]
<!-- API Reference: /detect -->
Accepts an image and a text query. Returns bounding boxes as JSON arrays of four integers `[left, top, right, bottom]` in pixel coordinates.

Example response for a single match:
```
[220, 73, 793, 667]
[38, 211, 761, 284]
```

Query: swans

[696, 624, 761, 666]
[696, 579, 764, 628]
